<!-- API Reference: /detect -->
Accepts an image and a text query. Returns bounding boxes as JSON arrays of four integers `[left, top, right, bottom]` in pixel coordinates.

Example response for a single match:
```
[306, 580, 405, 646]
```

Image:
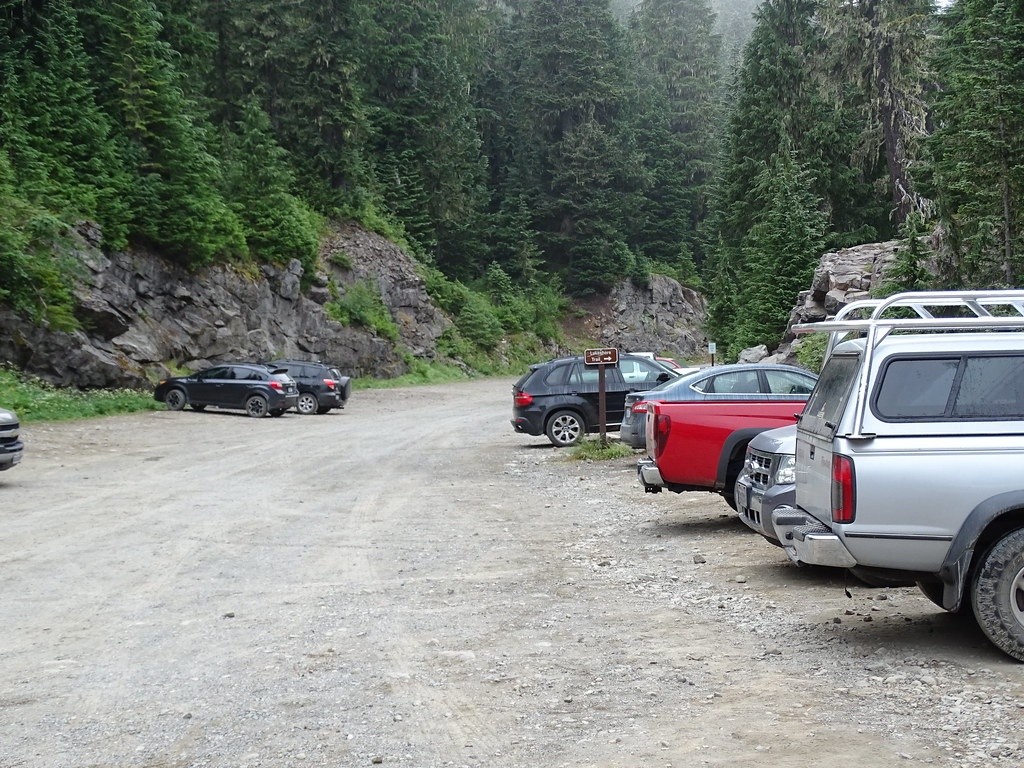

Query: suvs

[769, 286, 1024, 662]
[732, 423, 799, 549]
[269, 361, 351, 415]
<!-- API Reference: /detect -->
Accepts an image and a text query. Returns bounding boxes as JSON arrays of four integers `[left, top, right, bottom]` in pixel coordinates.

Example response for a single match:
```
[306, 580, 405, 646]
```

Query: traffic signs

[583, 347, 619, 365]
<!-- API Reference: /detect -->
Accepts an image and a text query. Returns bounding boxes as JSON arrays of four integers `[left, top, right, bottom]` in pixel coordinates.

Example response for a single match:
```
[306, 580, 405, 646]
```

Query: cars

[509, 352, 702, 447]
[618, 362, 819, 450]
[152, 361, 300, 418]
[0, 407, 24, 471]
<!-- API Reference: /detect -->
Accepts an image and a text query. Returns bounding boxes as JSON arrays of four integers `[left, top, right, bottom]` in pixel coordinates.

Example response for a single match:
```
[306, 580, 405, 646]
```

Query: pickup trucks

[636, 398, 810, 512]
